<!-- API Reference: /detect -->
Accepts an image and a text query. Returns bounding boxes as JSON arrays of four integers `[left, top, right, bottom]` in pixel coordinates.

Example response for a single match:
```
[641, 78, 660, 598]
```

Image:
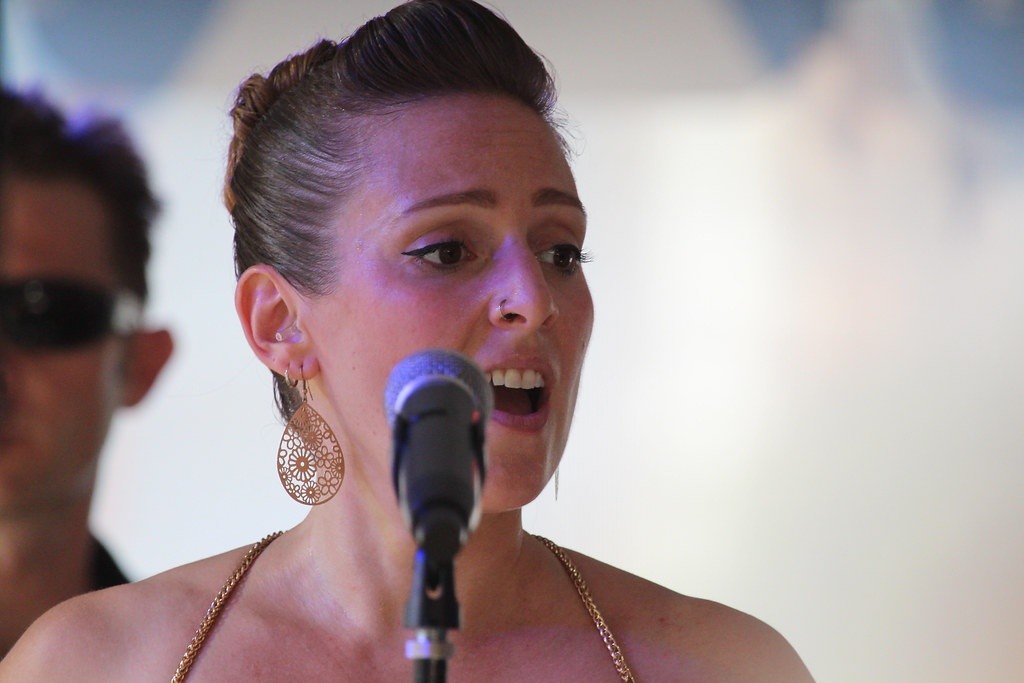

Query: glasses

[0, 275, 146, 354]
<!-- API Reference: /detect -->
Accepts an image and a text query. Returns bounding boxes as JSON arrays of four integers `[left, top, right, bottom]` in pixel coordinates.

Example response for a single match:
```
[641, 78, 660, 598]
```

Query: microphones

[382, 348, 493, 561]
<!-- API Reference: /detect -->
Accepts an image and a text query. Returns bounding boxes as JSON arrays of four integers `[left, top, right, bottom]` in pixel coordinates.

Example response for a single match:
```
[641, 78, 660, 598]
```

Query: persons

[0, 1, 821, 683]
[0, 88, 174, 658]
